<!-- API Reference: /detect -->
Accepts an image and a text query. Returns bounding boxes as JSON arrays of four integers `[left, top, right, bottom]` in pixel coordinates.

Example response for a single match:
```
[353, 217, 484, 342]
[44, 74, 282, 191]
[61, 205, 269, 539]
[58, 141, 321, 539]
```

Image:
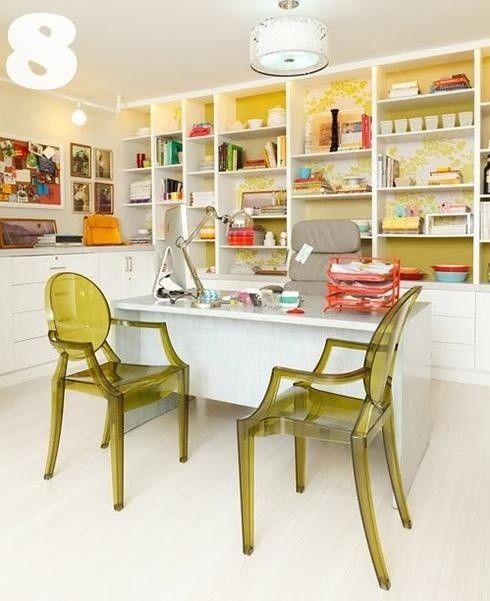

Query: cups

[164, 177, 183, 200]
[136, 153, 145, 168]
[298, 166, 312, 179]
[229, 207, 261, 218]
[143, 160, 151, 167]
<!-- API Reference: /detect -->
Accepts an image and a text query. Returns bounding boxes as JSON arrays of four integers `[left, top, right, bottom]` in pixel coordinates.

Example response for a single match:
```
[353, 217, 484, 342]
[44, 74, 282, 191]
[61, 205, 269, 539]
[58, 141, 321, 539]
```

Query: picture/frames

[70, 143, 113, 215]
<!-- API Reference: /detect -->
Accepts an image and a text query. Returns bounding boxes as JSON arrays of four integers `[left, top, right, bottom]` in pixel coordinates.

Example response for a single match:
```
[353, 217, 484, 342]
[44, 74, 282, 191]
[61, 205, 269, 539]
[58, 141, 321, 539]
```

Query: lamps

[249, 0, 329, 75]
[71, 102, 85, 126]
[175, 206, 254, 309]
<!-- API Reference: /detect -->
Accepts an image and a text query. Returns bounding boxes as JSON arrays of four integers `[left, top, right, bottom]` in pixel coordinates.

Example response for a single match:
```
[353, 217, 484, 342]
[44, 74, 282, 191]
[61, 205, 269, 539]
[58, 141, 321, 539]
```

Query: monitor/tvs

[152, 204, 194, 301]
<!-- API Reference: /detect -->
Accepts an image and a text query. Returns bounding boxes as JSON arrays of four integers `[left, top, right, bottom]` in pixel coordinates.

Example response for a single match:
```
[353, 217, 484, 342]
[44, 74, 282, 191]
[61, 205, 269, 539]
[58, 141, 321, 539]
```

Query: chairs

[283, 219, 363, 295]
[44, 273, 190, 510]
[237, 284, 422, 590]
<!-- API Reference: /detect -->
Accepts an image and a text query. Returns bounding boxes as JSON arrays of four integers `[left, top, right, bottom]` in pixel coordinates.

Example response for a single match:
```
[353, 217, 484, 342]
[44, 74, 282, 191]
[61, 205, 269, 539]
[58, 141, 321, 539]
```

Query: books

[33, 233, 84, 247]
[154, 136, 182, 166]
[328, 261, 399, 311]
[383, 73, 472, 102]
[376, 153, 471, 235]
[293, 177, 371, 196]
[480, 202, 490, 241]
[218, 136, 287, 172]
[258, 206, 285, 218]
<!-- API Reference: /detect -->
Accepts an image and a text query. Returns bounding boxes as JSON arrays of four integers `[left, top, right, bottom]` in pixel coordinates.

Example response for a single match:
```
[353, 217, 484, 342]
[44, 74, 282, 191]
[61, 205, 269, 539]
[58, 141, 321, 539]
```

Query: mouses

[222, 295, 231, 301]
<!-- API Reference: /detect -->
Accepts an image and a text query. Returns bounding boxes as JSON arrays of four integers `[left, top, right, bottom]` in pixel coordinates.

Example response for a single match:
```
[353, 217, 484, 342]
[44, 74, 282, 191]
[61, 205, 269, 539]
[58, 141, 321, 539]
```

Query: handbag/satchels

[82, 209, 122, 246]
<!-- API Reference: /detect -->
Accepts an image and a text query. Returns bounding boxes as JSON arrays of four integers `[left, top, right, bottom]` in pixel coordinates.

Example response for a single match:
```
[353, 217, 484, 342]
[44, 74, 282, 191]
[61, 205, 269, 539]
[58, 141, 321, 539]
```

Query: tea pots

[229, 120, 248, 131]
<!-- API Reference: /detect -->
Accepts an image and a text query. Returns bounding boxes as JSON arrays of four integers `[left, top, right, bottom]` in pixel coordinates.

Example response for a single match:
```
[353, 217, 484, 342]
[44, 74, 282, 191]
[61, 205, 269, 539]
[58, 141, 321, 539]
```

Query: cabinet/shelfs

[0, 252, 100, 390]
[399, 287, 490, 387]
[100, 249, 160, 364]
[123, 38, 488, 285]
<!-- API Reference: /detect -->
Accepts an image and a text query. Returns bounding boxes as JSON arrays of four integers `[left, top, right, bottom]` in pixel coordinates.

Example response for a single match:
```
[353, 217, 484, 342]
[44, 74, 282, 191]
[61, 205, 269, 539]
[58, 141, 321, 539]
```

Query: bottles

[484, 155, 490, 195]
[274, 189, 287, 206]
[487, 263, 490, 282]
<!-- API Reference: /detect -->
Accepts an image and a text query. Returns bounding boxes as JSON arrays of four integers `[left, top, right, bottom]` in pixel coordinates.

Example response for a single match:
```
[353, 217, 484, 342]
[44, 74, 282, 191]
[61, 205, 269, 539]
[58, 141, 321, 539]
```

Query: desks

[111, 289, 434, 507]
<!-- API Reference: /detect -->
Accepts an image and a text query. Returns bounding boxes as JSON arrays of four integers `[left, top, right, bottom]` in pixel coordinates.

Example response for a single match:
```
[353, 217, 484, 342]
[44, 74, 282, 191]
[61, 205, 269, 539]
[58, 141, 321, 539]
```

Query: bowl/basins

[248, 118, 263, 129]
[400, 267, 426, 280]
[429, 264, 471, 282]
